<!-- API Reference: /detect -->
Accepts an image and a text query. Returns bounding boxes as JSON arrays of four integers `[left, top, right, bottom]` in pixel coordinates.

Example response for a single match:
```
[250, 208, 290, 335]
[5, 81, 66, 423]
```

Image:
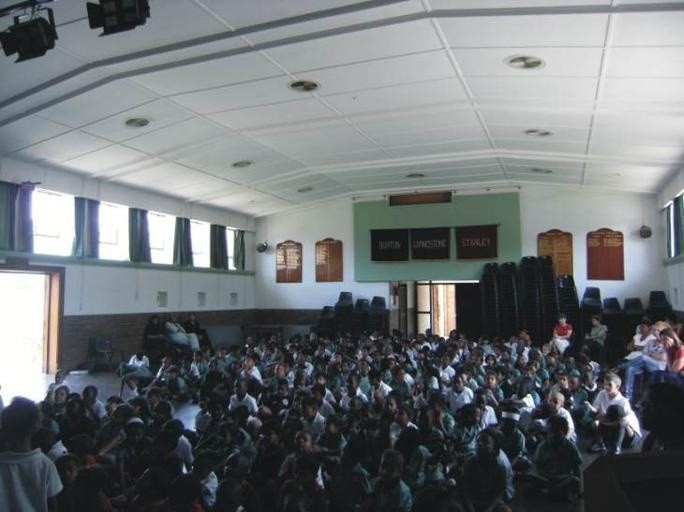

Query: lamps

[0, 0, 151, 64]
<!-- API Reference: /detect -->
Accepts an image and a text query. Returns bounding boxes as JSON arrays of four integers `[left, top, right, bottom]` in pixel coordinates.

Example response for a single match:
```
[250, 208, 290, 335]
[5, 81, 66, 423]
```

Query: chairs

[92, 336, 117, 372]
[478, 255, 676, 366]
[316, 291, 390, 340]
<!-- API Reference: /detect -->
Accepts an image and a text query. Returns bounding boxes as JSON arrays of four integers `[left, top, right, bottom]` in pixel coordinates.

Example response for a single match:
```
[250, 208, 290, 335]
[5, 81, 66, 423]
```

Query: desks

[243, 324, 287, 342]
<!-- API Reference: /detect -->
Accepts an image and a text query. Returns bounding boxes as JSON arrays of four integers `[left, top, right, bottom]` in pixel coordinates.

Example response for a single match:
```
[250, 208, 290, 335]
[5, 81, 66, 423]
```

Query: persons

[0, 312, 683, 511]
